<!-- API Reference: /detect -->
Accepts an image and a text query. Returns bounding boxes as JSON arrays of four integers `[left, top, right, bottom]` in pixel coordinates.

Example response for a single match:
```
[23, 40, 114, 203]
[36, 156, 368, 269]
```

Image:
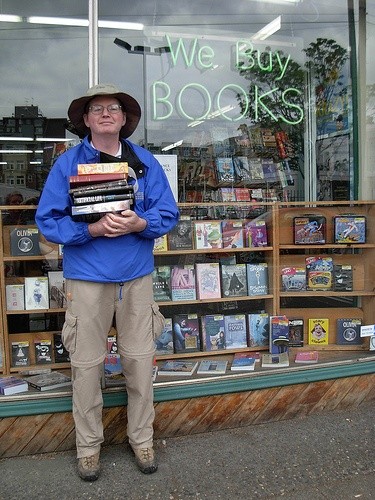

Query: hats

[68, 83, 142, 139]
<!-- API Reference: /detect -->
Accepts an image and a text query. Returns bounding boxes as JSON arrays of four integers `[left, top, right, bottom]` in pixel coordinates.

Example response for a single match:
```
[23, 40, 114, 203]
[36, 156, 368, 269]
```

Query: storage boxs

[200, 314, 226, 352]
[194, 263, 221, 300]
[293, 215, 327, 246]
[10, 227, 41, 257]
[232, 157, 251, 184]
[219, 264, 248, 298]
[243, 220, 268, 248]
[5, 284, 25, 311]
[217, 188, 239, 216]
[24, 277, 50, 310]
[192, 221, 223, 249]
[214, 157, 235, 184]
[172, 314, 201, 354]
[332, 214, 367, 244]
[246, 263, 269, 296]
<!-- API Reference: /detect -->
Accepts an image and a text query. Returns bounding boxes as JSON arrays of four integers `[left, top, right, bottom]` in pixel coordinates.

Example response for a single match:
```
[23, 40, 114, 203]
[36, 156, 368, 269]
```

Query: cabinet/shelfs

[0, 123, 375, 380]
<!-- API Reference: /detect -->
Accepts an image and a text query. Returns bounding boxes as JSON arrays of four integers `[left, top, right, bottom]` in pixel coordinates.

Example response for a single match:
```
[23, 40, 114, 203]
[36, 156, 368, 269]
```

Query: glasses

[87, 104, 122, 115]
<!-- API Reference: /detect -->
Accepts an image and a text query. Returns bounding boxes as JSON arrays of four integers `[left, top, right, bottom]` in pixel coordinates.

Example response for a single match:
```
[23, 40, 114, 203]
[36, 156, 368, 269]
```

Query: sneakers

[132, 445, 159, 474]
[78, 452, 101, 482]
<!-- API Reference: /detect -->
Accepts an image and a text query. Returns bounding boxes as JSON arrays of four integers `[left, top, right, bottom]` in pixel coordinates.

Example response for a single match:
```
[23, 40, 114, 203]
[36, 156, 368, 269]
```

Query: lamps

[0, 0, 145, 31]
[143, 7, 304, 49]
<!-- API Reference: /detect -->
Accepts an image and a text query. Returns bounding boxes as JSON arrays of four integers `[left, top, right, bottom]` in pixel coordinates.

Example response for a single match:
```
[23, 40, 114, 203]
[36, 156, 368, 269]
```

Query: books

[0, 127, 375, 397]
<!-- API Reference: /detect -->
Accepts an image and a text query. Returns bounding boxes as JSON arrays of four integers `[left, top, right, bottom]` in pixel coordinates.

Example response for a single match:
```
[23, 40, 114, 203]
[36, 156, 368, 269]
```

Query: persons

[2, 192, 44, 278]
[34, 84, 180, 483]
